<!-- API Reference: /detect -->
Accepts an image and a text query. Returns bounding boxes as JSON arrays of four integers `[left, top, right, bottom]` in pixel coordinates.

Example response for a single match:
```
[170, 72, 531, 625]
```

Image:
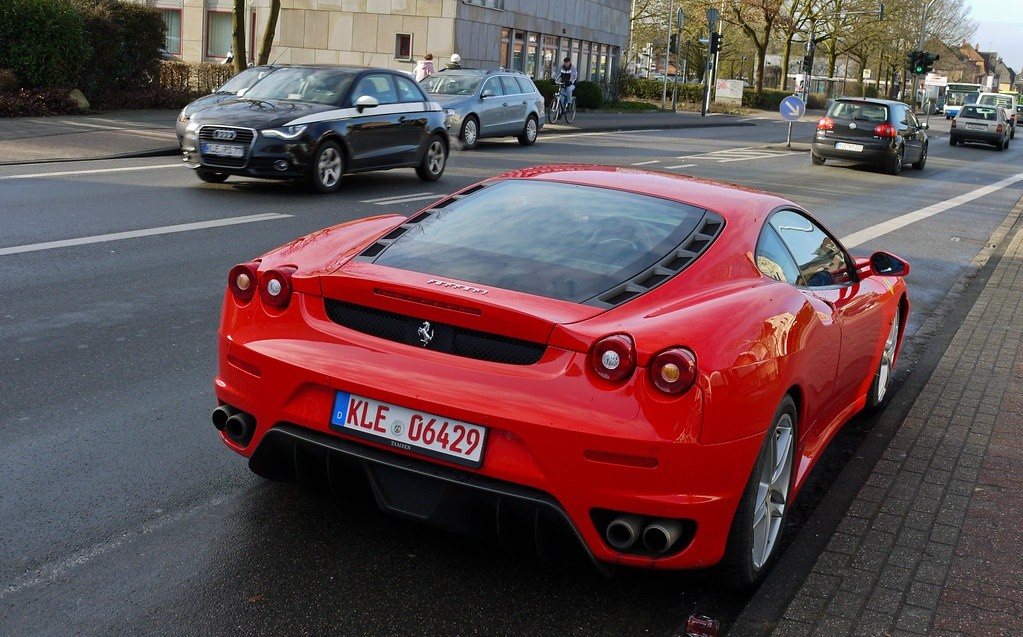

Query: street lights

[802, 4, 884, 107]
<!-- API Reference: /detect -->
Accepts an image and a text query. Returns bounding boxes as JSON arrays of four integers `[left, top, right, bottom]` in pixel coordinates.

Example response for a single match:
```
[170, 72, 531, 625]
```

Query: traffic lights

[915, 52, 926, 74]
[712, 32, 723, 53]
[670, 34, 676, 53]
[906, 51, 915, 73]
[927, 53, 940, 72]
[803, 56, 811, 72]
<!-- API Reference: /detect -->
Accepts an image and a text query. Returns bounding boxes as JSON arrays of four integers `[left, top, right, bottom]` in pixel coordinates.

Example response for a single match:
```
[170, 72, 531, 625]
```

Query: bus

[943, 82, 988, 121]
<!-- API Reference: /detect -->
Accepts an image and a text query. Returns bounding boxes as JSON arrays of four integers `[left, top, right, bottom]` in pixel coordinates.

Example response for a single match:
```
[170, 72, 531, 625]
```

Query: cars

[950, 104, 1012, 151]
[811, 97, 929, 175]
[636, 72, 704, 84]
[175, 63, 452, 194]
[1017, 105, 1023, 124]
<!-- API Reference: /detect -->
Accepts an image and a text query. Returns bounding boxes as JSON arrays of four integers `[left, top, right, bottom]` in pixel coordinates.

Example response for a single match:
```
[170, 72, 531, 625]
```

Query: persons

[221, 44, 234, 65]
[414, 53, 434, 82]
[445, 53, 461, 68]
[552, 57, 578, 119]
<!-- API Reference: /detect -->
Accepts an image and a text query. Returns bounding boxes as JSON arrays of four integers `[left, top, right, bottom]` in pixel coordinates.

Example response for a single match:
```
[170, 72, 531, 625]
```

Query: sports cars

[212, 164, 912, 593]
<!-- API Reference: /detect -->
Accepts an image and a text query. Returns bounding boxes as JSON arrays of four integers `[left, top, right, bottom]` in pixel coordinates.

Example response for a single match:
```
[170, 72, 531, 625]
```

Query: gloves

[552, 80, 557, 85]
[565, 81, 572, 87]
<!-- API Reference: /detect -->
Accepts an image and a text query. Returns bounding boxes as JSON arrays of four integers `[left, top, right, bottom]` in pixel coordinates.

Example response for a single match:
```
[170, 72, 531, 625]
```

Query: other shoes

[554, 115, 561, 120]
[567, 103, 573, 111]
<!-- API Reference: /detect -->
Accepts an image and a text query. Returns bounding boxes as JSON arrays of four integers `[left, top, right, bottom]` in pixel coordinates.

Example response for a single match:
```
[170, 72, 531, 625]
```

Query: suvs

[406, 67, 546, 149]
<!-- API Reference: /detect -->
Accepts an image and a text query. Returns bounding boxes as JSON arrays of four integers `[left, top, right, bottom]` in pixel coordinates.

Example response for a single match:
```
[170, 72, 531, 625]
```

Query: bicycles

[548, 80, 577, 124]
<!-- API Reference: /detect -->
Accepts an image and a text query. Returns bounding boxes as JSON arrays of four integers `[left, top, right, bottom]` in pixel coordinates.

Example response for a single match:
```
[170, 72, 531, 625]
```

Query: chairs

[847, 108, 862, 118]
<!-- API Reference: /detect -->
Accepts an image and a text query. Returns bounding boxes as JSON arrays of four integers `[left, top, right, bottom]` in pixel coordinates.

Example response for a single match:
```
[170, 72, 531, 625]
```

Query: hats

[450, 54, 460, 62]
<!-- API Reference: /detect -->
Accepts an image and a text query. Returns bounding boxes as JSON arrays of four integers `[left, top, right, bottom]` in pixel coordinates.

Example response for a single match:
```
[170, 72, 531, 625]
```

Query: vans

[976, 92, 1017, 139]
